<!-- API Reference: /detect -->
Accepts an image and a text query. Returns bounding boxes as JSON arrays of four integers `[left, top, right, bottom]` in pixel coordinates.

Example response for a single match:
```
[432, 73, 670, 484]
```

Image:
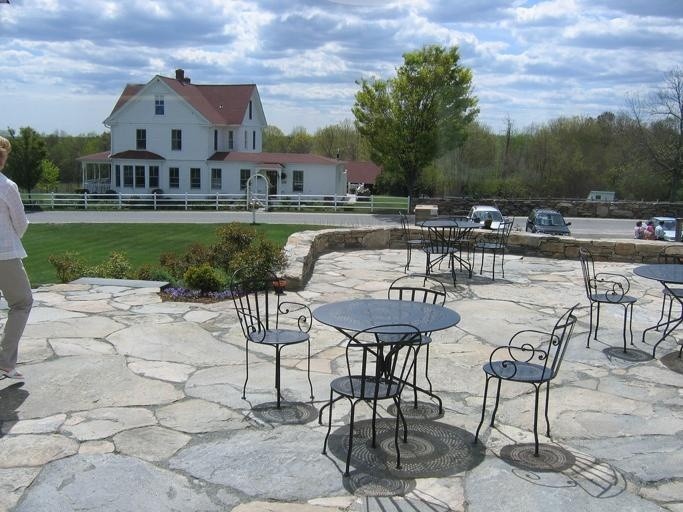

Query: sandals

[0, 367, 24, 380]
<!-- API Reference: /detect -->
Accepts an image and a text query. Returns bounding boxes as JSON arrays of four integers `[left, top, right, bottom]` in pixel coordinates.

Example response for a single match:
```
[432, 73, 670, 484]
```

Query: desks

[312, 299, 460, 414]
[633, 264, 683, 358]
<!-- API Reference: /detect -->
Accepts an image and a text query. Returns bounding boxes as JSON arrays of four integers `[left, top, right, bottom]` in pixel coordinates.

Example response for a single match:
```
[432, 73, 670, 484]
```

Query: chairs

[475, 302, 580, 457]
[399, 209, 514, 289]
[322, 324, 422, 476]
[655, 244, 683, 340]
[382, 274, 446, 409]
[579, 246, 636, 354]
[229, 265, 314, 410]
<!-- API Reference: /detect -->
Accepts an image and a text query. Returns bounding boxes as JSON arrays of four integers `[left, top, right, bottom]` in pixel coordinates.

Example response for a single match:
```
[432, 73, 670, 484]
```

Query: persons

[655, 221, 666, 240]
[633, 221, 645, 239]
[646, 221, 656, 240]
[0, 134, 34, 381]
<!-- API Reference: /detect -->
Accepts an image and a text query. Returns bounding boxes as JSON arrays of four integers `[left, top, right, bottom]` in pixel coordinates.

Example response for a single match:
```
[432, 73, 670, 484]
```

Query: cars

[646, 216, 682, 241]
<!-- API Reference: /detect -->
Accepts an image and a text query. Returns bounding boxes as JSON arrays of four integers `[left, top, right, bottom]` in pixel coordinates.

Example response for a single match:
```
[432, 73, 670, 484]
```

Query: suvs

[466, 205, 505, 230]
[525, 206, 571, 237]
